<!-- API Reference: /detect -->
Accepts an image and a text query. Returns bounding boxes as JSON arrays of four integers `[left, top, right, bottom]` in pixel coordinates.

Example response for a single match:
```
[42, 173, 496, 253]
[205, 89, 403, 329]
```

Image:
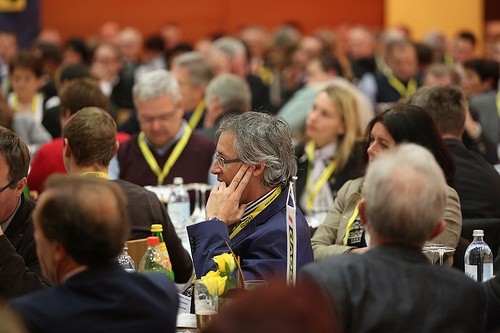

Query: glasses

[215, 152, 242, 167]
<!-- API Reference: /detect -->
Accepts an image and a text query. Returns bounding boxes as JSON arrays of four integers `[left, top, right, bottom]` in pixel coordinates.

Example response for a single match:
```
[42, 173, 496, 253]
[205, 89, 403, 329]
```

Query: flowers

[200, 252, 236, 298]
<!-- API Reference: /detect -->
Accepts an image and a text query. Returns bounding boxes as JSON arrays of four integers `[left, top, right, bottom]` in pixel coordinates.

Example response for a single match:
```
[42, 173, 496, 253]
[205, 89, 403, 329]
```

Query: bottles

[150, 223, 175, 282]
[117, 243, 136, 273]
[464, 229, 495, 283]
[138, 237, 169, 275]
[168, 176, 190, 237]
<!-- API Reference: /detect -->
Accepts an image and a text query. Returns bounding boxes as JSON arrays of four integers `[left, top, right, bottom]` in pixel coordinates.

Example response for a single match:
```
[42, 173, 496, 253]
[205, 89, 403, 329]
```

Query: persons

[1, 21, 500, 165]
[186, 111, 314, 291]
[309, 105, 464, 263]
[6, 174, 179, 333]
[293, 82, 370, 234]
[0, 127, 52, 300]
[107, 71, 223, 187]
[298, 144, 489, 332]
[26, 78, 134, 199]
[61, 107, 193, 285]
[409, 84, 500, 267]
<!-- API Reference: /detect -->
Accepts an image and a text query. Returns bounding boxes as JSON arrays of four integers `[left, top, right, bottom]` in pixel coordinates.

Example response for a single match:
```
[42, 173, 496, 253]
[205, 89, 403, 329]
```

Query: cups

[194, 279, 219, 331]
[245, 279, 268, 291]
[421, 242, 456, 269]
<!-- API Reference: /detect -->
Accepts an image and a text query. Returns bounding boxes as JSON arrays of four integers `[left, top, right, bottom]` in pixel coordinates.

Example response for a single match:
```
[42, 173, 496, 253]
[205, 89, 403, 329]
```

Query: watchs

[207, 215, 224, 222]
